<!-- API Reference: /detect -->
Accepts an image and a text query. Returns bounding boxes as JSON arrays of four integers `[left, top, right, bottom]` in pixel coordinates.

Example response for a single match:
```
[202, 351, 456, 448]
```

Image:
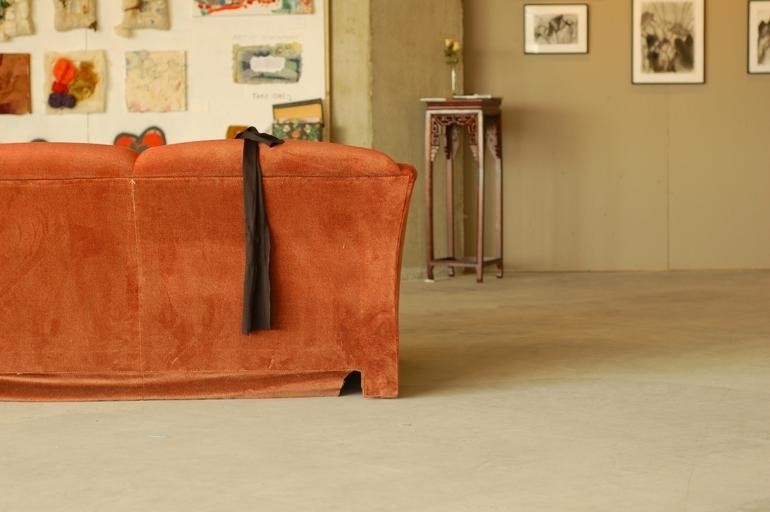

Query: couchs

[1, 138, 416, 401]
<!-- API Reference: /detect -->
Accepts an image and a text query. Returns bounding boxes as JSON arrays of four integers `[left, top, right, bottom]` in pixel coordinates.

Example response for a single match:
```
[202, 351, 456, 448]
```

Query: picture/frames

[631, 1, 705, 85]
[522, 3, 590, 56]
[746, 1, 770, 75]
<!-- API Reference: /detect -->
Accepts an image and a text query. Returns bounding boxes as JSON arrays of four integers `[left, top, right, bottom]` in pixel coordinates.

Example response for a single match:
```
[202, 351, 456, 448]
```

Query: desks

[420, 94, 506, 284]
